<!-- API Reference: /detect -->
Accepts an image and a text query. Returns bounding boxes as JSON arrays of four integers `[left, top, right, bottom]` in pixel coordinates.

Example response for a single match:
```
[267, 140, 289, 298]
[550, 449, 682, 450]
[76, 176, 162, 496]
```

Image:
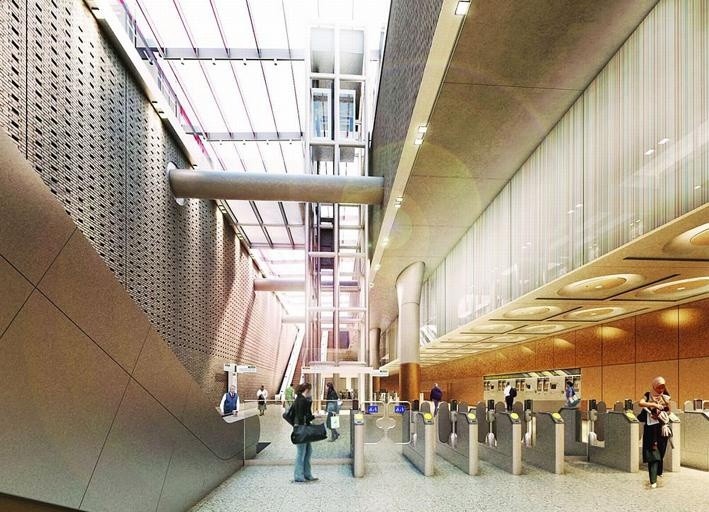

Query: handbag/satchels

[330, 413, 340, 429]
[566, 389, 575, 397]
[283, 405, 294, 427]
[291, 423, 327, 444]
[637, 409, 647, 422]
[259, 394, 265, 405]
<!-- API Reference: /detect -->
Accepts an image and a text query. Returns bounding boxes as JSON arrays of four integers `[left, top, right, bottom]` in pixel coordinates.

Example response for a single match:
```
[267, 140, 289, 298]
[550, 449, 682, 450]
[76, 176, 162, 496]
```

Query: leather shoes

[295, 478, 318, 482]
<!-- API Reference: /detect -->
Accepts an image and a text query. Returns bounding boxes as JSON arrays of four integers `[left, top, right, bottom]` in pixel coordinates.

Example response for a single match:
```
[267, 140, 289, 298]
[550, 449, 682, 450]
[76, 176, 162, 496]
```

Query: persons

[430, 382, 442, 418]
[324, 381, 340, 441]
[566, 381, 575, 407]
[504, 382, 513, 411]
[294, 382, 319, 482]
[639, 375, 672, 488]
[256, 385, 268, 416]
[339, 391, 343, 399]
[220, 385, 240, 416]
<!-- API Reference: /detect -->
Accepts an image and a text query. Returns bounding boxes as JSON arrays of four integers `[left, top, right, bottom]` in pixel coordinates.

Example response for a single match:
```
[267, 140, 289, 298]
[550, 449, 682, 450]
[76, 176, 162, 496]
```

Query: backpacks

[510, 387, 517, 397]
